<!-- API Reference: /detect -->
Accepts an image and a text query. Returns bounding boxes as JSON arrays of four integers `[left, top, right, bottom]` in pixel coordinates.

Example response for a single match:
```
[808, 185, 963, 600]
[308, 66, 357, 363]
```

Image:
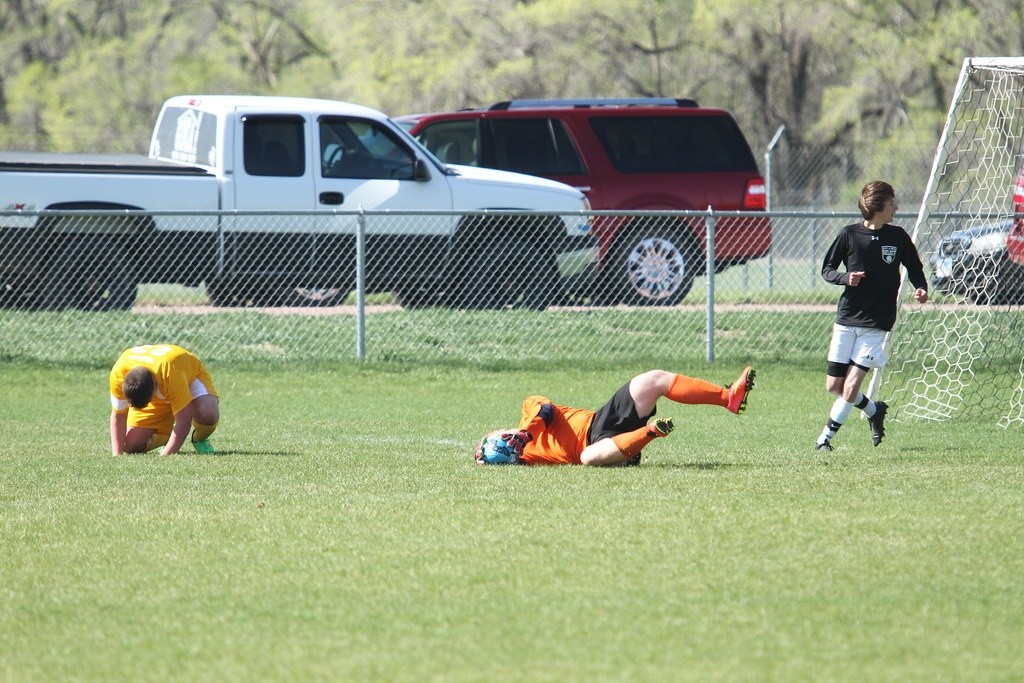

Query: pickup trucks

[0, 95, 596, 313]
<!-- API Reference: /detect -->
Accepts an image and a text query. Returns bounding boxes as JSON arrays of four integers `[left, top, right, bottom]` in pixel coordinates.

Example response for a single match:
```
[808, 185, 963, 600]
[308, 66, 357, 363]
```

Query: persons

[110, 344, 220, 456]
[474, 366, 755, 467]
[815, 181, 927, 452]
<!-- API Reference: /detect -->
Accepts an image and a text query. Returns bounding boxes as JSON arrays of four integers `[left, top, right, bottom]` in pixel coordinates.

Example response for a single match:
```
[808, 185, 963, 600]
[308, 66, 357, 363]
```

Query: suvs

[359, 98, 771, 309]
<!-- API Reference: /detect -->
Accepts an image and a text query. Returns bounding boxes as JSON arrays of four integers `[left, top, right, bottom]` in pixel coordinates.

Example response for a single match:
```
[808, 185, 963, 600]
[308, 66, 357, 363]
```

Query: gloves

[500, 429, 533, 456]
[475, 434, 490, 466]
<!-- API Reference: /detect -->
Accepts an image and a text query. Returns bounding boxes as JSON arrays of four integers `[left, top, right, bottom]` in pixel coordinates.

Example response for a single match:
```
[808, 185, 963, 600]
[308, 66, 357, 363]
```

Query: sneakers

[647, 417, 676, 438]
[866, 401, 889, 447]
[191, 429, 216, 456]
[816, 439, 835, 453]
[724, 366, 756, 416]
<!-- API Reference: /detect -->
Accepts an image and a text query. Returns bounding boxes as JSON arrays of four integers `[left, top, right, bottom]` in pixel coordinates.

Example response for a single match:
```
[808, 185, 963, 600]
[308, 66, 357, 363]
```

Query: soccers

[482, 431, 520, 465]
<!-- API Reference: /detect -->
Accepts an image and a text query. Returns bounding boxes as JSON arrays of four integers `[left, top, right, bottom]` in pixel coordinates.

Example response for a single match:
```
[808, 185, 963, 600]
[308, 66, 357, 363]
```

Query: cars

[930, 220, 1024, 304]
